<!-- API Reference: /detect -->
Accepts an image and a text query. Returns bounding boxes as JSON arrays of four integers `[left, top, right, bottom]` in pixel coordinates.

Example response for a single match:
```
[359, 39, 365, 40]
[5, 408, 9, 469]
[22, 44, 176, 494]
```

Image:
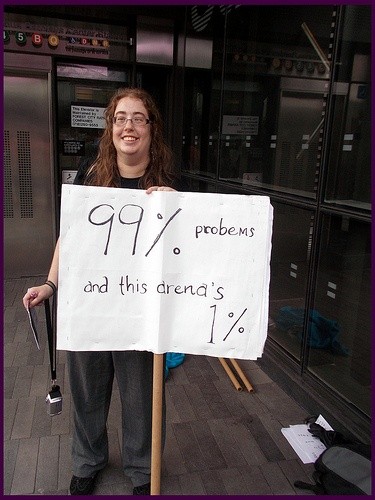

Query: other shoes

[133, 482, 151, 495]
[70, 468, 103, 495]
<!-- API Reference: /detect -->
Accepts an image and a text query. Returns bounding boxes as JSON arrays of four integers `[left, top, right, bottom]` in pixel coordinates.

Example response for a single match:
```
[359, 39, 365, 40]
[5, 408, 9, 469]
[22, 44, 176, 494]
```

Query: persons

[23, 87, 200, 495]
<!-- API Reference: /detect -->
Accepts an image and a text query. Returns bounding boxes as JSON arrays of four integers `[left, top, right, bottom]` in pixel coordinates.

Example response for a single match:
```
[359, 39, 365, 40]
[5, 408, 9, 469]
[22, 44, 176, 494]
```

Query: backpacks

[295, 423, 371, 495]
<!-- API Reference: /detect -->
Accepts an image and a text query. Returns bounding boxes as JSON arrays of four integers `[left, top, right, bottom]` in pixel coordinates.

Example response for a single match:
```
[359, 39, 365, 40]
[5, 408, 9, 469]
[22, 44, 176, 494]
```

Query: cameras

[45, 390, 63, 417]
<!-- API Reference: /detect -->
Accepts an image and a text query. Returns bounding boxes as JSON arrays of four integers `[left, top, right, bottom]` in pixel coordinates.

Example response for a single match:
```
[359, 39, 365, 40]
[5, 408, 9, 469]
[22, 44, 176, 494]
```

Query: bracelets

[43, 279, 57, 295]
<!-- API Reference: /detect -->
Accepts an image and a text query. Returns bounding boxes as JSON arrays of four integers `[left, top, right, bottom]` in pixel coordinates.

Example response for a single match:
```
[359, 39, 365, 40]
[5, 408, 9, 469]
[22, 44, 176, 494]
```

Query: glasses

[112, 116, 151, 126]
